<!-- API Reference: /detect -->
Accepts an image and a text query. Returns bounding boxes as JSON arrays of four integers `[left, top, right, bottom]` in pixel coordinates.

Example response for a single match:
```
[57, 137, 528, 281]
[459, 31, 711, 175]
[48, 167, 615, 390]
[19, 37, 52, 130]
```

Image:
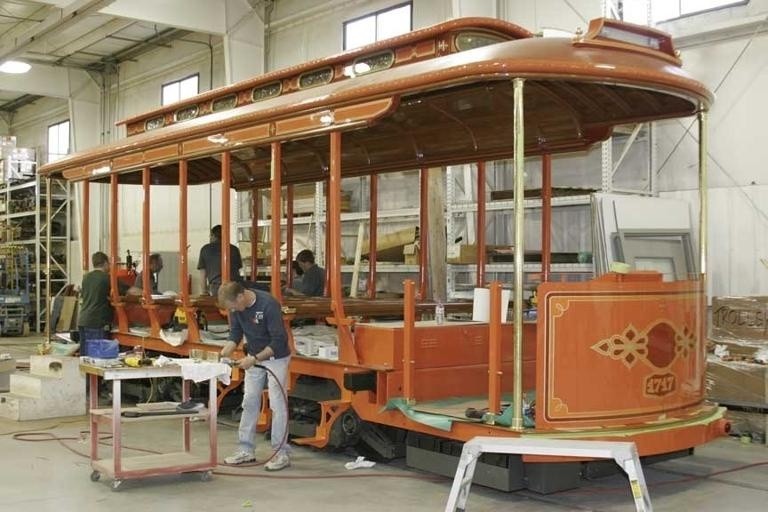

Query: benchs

[443, 434, 653, 511]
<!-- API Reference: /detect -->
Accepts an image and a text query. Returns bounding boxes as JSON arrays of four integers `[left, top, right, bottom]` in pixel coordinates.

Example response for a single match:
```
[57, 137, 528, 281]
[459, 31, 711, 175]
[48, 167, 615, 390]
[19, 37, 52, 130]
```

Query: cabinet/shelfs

[78, 362, 219, 489]
[0, 178, 73, 335]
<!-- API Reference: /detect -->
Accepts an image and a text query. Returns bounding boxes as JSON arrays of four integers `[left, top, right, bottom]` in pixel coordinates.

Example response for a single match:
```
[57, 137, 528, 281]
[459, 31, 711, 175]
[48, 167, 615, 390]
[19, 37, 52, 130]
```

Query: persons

[196, 225, 243, 297]
[212, 280, 291, 471]
[77, 251, 131, 402]
[134, 253, 166, 295]
[280, 247, 328, 328]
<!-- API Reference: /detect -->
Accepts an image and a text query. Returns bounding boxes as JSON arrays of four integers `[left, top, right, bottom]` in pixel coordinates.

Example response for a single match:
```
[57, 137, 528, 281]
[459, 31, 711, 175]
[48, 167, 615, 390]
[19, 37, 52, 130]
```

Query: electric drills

[119, 358, 152, 368]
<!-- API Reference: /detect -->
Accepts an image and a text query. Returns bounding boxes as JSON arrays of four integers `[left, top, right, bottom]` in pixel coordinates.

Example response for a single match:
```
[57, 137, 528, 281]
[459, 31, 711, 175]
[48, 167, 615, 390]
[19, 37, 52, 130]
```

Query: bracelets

[253, 354, 259, 363]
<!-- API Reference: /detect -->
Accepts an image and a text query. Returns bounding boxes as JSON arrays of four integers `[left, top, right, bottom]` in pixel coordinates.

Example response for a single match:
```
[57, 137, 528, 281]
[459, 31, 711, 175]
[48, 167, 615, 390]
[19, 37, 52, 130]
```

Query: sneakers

[264, 454, 290, 472]
[223, 451, 256, 465]
